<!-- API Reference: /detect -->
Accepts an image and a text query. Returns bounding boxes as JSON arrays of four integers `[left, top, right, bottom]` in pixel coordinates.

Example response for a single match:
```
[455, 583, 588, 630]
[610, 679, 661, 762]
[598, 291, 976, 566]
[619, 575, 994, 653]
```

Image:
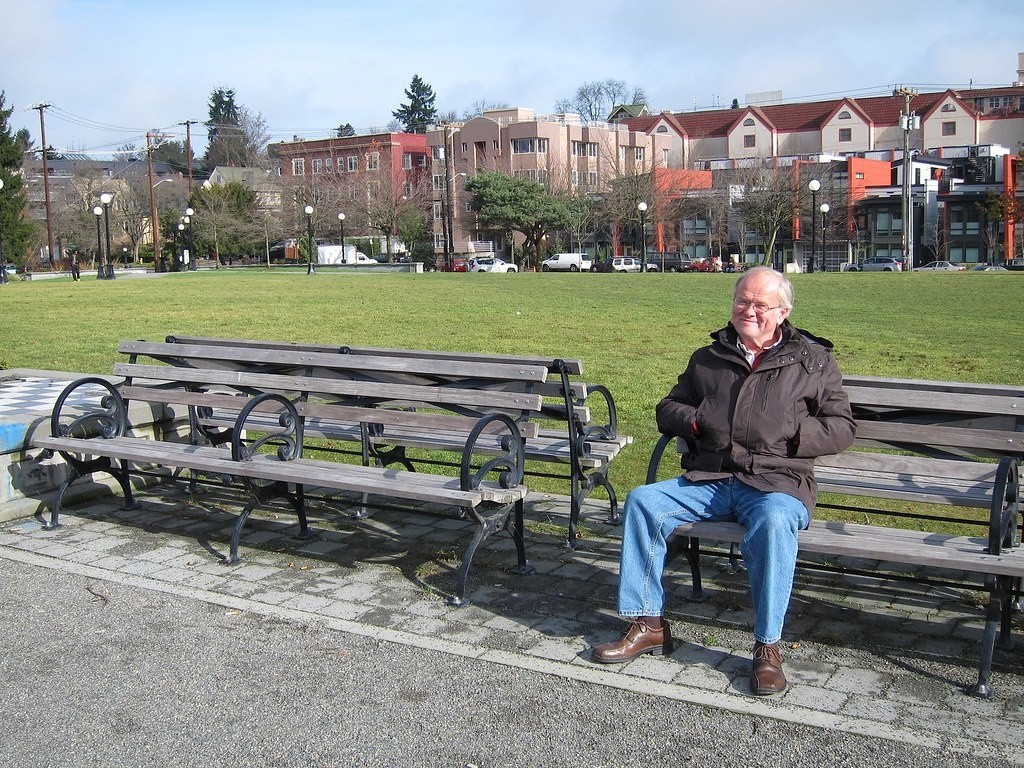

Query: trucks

[316, 244, 378, 268]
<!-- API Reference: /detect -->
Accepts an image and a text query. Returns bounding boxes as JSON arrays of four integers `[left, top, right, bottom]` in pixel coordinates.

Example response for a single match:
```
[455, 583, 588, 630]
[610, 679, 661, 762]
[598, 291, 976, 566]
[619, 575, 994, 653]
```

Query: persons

[590, 265, 859, 692]
[726, 257, 736, 273]
[714, 256, 723, 273]
[68, 250, 80, 282]
[219, 252, 226, 265]
[0, 265, 8, 284]
[211, 249, 217, 260]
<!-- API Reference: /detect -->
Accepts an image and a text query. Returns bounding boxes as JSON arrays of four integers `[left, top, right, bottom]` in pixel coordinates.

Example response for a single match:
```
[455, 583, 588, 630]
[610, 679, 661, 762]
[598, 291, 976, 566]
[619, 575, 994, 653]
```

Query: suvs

[467, 257, 519, 273]
[647, 251, 692, 273]
[843, 256, 902, 272]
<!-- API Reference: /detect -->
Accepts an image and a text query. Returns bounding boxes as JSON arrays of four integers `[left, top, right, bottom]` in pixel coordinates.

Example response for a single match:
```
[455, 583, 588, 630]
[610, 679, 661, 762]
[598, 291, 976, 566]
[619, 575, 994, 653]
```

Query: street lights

[807, 179, 821, 273]
[337, 212, 346, 264]
[183, 215, 192, 271]
[123, 247, 128, 268]
[638, 201, 648, 274]
[178, 224, 187, 272]
[446, 173, 467, 273]
[94, 206, 106, 280]
[304, 205, 316, 275]
[819, 203, 830, 272]
[185, 208, 197, 271]
[100, 193, 116, 280]
[150, 179, 174, 273]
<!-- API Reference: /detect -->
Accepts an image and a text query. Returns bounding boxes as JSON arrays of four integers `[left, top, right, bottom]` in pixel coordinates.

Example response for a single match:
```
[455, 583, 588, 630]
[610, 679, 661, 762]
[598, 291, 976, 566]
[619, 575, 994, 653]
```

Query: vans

[541, 253, 593, 272]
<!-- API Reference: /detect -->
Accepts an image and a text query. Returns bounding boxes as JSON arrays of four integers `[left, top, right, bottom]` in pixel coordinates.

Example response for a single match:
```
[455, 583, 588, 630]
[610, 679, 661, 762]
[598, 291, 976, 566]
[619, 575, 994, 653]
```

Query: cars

[440, 258, 469, 272]
[370, 252, 438, 273]
[590, 256, 661, 273]
[689, 257, 729, 273]
[269, 240, 303, 260]
[912, 260, 967, 272]
[969, 265, 1008, 272]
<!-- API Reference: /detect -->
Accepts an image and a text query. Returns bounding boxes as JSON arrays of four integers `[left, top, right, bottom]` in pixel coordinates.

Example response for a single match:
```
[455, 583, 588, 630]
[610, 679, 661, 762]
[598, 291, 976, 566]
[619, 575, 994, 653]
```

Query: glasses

[732, 296, 782, 314]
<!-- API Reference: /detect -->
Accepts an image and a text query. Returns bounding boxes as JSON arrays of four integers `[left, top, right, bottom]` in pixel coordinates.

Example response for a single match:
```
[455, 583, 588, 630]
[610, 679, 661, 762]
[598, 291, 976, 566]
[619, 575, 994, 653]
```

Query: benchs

[645, 374, 1024, 698]
[32, 334, 633, 608]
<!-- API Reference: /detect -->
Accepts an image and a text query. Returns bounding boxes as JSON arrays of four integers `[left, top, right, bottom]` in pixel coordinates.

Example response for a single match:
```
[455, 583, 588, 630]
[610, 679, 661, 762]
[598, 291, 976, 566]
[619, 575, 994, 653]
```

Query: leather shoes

[750, 644, 786, 695]
[592, 621, 673, 663]
